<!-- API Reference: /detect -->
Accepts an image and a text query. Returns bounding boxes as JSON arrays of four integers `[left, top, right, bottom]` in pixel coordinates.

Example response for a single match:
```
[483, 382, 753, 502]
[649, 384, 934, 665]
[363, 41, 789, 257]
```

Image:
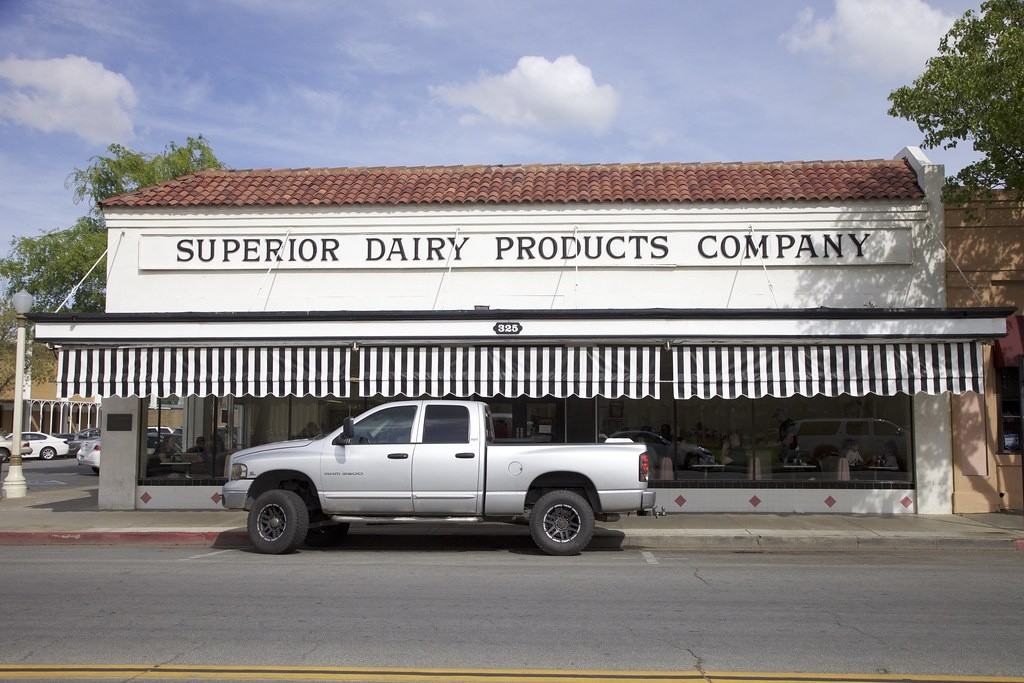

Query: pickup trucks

[221, 399, 655, 557]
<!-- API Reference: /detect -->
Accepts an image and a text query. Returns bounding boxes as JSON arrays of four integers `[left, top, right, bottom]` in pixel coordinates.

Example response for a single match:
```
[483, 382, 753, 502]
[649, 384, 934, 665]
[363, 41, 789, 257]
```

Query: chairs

[181, 453, 212, 475]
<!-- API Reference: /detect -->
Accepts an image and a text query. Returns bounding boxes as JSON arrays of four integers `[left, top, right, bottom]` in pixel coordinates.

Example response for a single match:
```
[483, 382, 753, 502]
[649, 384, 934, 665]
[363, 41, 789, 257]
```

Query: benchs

[822, 455, 860, 481]
[747, 456, 772, 480]
[653, 455, 675, 481]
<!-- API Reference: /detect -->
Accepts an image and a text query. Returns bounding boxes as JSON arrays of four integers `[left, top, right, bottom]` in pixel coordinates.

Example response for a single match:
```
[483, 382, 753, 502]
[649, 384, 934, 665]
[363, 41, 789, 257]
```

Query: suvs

[607, 431, 716, 472]
[785, 417, 908, 472]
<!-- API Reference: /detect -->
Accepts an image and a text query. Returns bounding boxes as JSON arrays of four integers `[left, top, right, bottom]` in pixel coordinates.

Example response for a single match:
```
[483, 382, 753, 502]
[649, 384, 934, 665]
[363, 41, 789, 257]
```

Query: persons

[147, 433, 225, 484]
[640, 408, 902, 488]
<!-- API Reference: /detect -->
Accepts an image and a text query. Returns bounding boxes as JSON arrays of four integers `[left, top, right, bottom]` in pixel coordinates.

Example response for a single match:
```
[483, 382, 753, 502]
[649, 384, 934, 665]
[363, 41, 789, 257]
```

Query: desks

[864, 465, 898, 481]
[743, 443, 784, 470]
[160, 461, 191, 478]
[783, 464, 817, 489]
[691, 463, 726, 481]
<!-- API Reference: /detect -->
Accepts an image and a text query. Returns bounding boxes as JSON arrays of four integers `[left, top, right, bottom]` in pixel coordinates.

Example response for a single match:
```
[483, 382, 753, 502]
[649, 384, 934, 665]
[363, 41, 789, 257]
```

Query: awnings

[358, 345, 662, 400]
[55, 345, 351, 398]
[671, 341, 986, 400]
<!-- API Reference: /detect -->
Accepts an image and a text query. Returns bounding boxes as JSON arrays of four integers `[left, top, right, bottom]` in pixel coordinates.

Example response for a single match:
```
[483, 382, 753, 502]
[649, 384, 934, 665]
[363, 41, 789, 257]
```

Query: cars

[80, 427, 101, 439]
[145, 427, 183, 456]
[77, 439, 99, 475]
[0, 434, 33, 463]
[56, 433, 91, 459]
[5, 432, 69, 461]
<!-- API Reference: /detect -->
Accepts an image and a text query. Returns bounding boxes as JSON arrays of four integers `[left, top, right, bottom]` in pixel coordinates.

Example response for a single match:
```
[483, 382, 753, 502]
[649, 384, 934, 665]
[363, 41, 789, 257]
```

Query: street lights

[1, 287, 34, 501]
